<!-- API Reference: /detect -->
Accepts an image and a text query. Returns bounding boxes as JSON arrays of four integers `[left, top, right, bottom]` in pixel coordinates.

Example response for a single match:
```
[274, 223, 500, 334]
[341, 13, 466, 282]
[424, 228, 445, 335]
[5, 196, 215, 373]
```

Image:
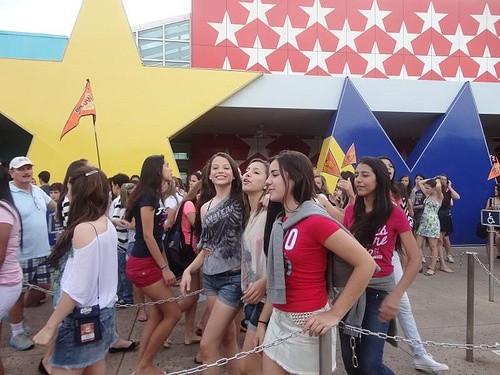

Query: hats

[9, 156, 35, 169]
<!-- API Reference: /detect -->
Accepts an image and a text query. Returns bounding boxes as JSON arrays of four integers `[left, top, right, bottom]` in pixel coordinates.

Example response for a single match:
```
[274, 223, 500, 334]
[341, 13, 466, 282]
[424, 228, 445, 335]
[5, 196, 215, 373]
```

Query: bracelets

[258, 321, 268, 325]
[161, 264, 168, 270]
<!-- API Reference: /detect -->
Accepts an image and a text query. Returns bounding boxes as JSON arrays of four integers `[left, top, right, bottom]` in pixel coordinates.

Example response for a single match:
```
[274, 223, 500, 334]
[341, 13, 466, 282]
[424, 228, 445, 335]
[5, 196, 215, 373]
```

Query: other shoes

[164, 340, 170, 347]
[418, 254, 454, 275]
[108, 339, 140, 353]
[497, 255, 500, 259]
[39, 358, 49, 375]
[185, 337, 201, 344]
[488, 262, 490, 267]
[138, 307, 147, 321]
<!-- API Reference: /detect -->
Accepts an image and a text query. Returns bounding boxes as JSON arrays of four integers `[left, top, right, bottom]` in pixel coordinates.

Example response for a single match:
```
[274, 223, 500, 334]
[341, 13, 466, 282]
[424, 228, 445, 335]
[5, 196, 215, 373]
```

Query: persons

[253, 150, 377, 375]
[32, 166, 119, 375]
[0, 149, 461, 375]
[485, 183, 500, 266]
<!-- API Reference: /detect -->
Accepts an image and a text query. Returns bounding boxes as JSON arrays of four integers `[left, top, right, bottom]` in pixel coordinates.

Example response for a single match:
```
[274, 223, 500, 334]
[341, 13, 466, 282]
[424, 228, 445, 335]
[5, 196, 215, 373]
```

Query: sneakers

[413, 353, 449, 371]
[9, 331, 34, 350]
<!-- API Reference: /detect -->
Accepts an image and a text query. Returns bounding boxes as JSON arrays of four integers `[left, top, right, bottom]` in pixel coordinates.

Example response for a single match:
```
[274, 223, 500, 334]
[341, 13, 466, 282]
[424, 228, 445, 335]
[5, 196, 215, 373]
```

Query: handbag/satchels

[74, 304, 103, 346]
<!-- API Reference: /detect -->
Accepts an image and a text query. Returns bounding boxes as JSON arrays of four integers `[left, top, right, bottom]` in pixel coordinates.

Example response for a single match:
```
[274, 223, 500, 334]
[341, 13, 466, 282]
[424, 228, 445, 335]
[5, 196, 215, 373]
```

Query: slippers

[194, 356, 203, 364]
[197, 328, 203, 336]
